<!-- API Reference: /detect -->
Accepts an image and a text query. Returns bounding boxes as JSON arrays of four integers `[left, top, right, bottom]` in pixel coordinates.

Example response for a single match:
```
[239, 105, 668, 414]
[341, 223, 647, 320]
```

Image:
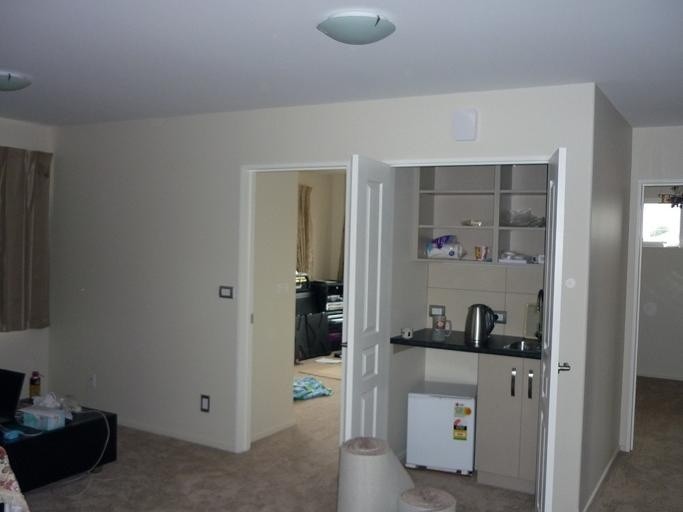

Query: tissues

[21, 393, 67, 431]
[426, 242, 463, 260]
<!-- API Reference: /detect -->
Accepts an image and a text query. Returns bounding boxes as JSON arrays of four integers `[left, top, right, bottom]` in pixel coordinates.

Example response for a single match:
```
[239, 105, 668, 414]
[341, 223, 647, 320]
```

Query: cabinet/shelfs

[475, 353, 541, 497]
[414, 163, 548, 267]
[0, 399, 116, 493]
[310, 280, 343, 351]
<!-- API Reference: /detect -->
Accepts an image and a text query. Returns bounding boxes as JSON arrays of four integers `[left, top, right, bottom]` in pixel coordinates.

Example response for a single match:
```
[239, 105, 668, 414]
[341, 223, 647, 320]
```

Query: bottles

[28, 370, 40, 400]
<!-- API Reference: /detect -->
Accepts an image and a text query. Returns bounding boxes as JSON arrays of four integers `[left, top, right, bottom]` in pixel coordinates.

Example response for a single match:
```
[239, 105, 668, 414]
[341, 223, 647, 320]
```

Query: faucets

[536, 288, 544, 332]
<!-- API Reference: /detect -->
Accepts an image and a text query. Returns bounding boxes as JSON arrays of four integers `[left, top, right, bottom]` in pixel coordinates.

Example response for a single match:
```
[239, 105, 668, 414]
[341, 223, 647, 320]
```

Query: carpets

[299, 355, 343, 379]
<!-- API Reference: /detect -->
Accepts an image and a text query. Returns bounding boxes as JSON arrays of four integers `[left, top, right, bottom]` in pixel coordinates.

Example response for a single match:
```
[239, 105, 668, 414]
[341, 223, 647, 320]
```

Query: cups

[531, 254, 544, 264]
[431, 314, 453, 342]
[400, 327, 414, 341]
[474, 245, 486, 261]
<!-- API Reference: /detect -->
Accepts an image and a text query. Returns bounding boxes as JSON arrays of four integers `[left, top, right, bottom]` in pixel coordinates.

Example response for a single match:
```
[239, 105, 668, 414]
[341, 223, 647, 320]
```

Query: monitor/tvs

[0, 369, 26, 422]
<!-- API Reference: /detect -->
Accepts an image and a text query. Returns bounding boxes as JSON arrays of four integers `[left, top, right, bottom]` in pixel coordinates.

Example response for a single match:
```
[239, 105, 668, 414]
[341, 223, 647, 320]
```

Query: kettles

[463, 303, 495, 349]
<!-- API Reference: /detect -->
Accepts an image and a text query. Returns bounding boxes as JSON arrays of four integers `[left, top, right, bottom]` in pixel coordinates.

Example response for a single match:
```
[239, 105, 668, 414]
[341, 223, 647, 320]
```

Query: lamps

[0, 72, 31, 91]
[316, 11, 395, 45]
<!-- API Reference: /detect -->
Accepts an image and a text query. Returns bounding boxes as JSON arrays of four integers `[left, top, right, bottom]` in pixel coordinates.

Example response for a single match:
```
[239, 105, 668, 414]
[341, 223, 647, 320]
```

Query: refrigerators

[403, 379, 477, 478]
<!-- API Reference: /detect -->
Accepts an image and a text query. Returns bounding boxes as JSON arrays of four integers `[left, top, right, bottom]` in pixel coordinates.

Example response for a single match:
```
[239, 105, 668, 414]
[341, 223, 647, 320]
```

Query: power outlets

[200, 396, 210, 412]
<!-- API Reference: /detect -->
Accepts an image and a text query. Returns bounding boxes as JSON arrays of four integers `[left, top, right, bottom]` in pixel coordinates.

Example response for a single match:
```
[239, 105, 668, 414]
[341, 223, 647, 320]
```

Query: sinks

[502, 339, 542, 353]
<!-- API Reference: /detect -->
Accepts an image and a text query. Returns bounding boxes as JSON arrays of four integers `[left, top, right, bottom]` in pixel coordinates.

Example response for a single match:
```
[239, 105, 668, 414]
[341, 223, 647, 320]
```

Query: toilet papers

[337, 436, 416, 512]
[396, 486, 457, 512]
